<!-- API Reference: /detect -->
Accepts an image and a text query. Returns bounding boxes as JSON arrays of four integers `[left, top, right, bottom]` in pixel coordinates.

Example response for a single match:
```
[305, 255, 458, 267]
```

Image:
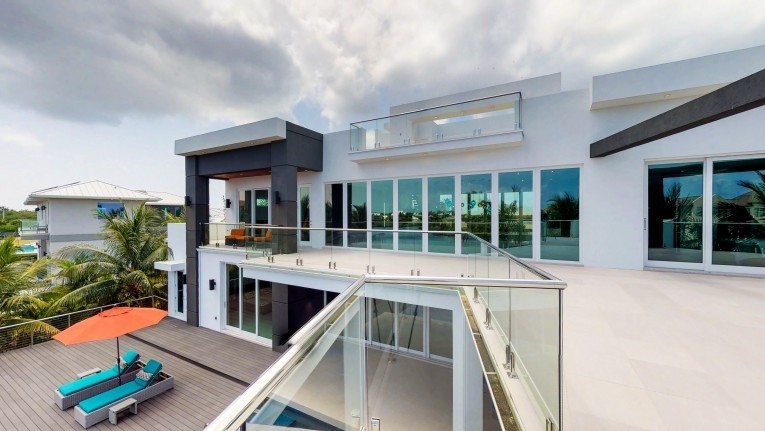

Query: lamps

[226, 198, 232, 208]
[209, 279, 216, 290]
[274, 191, 280, 204]
[185, 196, 191, 206]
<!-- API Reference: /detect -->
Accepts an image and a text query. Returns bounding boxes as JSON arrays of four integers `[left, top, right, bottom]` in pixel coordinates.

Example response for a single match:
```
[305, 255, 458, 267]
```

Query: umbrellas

[51, 304, 170, 386]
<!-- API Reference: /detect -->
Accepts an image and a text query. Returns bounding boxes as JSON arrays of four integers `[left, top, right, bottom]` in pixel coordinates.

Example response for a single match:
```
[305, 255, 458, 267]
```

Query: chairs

[54, 349, 145, 411]
[74, 359, 175, 428]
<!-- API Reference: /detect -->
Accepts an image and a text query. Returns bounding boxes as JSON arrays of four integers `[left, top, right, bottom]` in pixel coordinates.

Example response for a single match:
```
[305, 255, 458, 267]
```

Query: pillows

[117, 356, 126, 368]
[136, 370, 151, 380]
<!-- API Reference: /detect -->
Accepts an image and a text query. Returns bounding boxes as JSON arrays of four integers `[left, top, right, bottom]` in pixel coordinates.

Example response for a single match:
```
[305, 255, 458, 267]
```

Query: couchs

[225, 227, 272, 247]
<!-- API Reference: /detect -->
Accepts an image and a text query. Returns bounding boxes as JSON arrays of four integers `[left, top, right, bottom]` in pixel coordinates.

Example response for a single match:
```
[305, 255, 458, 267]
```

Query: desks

[77, 367, 102, 379]
[109, 398, 138, 425]
